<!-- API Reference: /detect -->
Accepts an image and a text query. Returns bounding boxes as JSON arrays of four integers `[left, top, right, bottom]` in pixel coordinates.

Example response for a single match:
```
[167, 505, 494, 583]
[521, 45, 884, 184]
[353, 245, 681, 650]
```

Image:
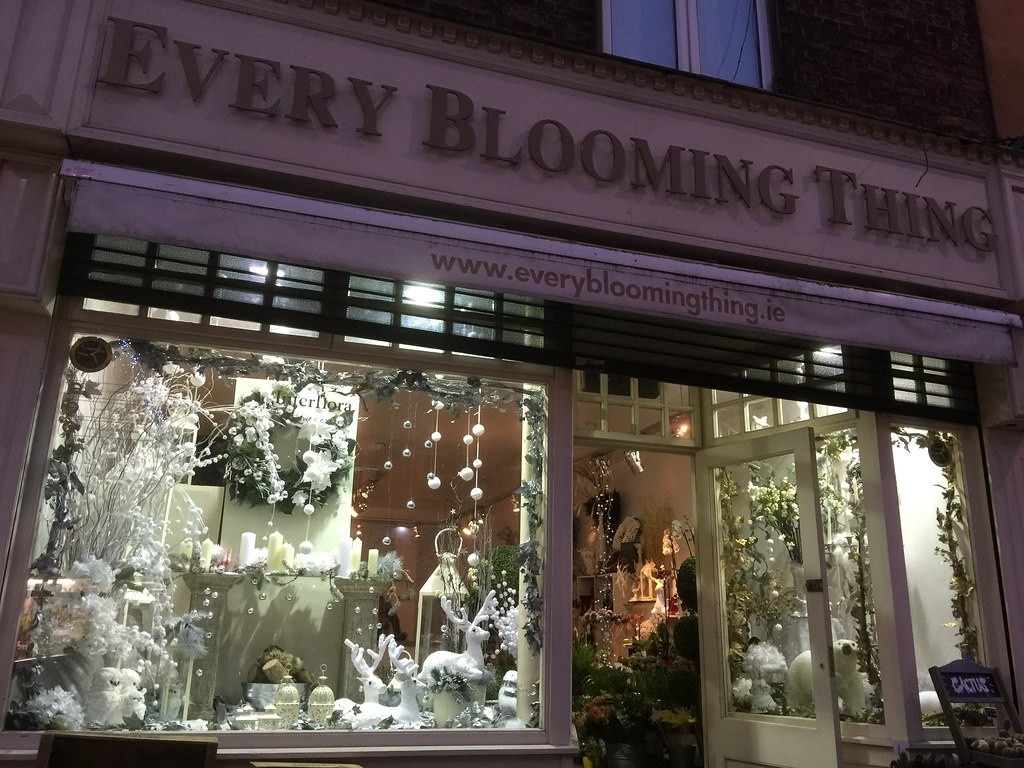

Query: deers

[333, 633, 426, 722]
[419, 590, 500, 679]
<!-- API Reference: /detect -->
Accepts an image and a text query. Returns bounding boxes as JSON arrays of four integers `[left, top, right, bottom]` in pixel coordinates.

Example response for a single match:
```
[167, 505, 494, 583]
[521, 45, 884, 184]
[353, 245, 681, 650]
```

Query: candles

[176, 530, 380, 582]
